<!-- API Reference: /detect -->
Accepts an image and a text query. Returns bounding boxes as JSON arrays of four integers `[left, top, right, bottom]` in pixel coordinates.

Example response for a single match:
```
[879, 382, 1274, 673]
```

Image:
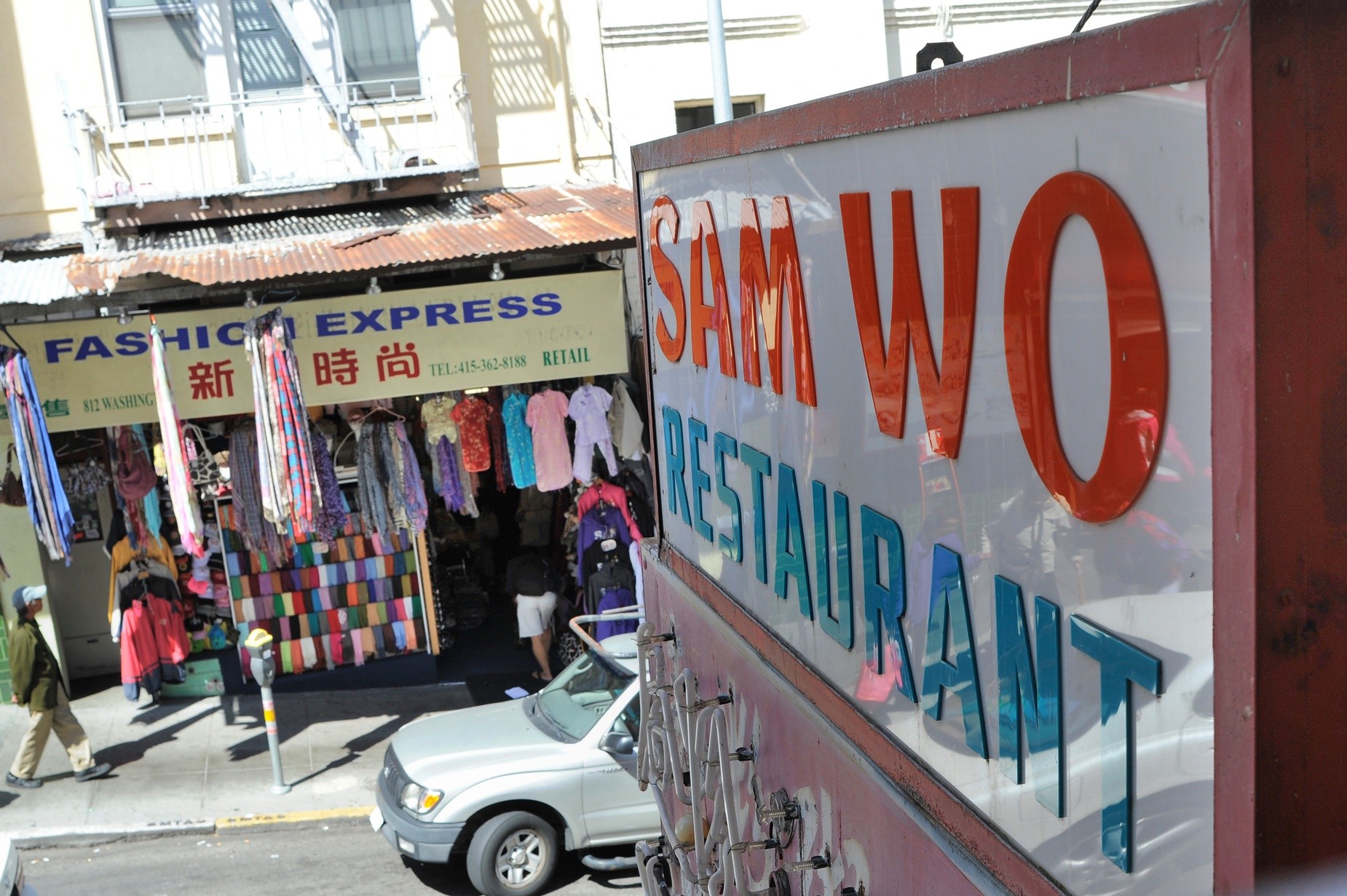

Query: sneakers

[5, 771, 43, 790]
[74, 761, 112, 783]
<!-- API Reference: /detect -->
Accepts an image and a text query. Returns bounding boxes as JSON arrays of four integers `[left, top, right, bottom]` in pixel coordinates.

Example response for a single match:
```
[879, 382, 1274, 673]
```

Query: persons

[503, 545, 565, 687]
[5, 583, 115, 791]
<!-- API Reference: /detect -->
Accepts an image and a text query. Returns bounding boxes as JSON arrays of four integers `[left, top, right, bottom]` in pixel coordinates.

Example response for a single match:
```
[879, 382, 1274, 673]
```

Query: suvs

[370, 626, 666, 895]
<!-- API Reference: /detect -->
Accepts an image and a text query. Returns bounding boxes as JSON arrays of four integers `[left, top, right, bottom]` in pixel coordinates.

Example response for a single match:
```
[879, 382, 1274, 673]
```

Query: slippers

[531, 668, 554, 687]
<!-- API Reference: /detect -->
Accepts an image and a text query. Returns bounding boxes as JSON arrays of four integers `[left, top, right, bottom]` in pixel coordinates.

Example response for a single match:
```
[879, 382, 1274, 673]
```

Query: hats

[12, 584, 46, 612]
